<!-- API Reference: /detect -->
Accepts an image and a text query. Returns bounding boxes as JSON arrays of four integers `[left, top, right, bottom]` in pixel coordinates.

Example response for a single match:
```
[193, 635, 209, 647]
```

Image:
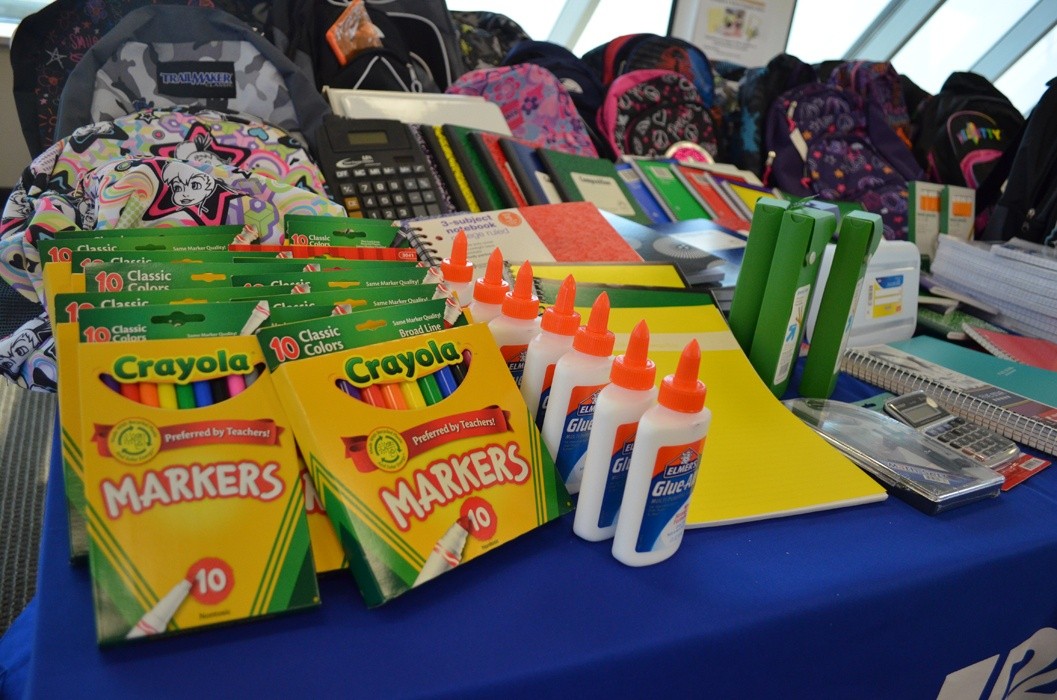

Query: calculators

[886, 390, 1019, 472]
[313, 117, 448, 222]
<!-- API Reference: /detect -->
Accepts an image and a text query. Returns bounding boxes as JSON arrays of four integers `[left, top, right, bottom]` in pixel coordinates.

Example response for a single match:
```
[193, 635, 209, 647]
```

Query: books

[399, 124, 1056, 531]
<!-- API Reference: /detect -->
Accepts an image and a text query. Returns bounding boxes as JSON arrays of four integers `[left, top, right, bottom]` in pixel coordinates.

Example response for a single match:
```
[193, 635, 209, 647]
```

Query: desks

[0, 327, 1057, 700]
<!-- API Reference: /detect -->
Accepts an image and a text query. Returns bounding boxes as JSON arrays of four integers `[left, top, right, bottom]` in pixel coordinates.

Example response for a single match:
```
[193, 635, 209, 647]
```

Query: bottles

[442, 227, 711, 569]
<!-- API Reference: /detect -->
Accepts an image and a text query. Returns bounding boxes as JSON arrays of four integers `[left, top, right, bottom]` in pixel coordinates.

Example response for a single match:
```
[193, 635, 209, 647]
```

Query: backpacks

[0, 0, 1056, 321]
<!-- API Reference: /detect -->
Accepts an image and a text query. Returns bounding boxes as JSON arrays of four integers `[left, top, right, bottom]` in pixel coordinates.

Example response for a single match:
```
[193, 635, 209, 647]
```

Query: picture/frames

[666, 1, 798, 81]
